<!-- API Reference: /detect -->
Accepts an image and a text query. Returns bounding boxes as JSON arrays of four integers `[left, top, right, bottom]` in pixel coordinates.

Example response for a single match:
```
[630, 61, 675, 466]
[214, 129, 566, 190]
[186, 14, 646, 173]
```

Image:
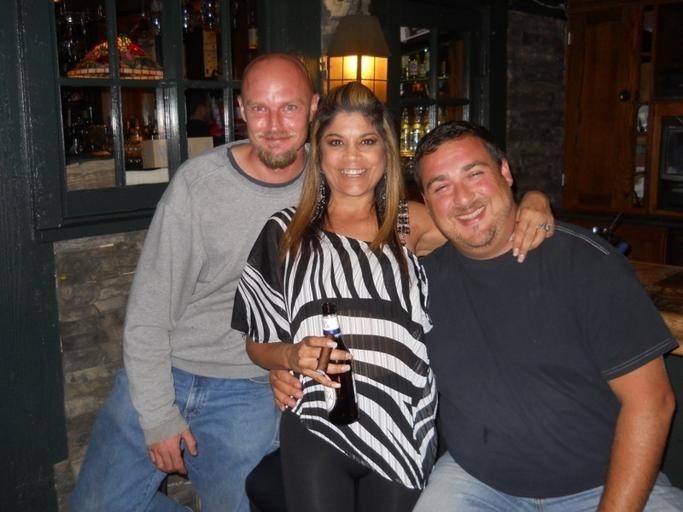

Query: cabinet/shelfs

[396, 23, 468, 181]
[599, 223, 683, 266]
[565, 1, 653, 212]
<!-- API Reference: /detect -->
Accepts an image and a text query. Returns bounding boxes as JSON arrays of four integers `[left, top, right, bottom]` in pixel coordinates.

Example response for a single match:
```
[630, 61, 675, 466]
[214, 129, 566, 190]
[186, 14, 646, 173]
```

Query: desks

[570, 260, 683, 357]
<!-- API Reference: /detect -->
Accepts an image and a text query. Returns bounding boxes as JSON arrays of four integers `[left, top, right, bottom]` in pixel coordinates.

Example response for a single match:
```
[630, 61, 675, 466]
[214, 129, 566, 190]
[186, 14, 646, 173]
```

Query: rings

[540, 224, 550, 231]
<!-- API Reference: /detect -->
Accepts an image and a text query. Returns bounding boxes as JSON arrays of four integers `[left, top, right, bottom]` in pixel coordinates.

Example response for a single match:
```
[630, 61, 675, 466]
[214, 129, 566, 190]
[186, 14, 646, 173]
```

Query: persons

[270, 119, 682, 511]
[231, 82, 555, 512]
[68, 52, 316, 511]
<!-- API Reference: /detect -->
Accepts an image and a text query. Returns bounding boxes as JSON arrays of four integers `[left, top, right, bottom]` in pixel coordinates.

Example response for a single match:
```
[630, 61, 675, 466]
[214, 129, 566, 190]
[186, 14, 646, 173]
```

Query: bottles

[398, 46, 452, 160]
[320, 301, 360, 427]
[64, 103, 161, 171]
[183, 0, 260, 81]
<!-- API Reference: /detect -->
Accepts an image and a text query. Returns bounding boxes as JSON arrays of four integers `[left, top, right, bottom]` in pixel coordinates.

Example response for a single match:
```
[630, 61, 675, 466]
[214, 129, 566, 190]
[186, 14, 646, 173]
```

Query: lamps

[327, 15, 389, 110]
